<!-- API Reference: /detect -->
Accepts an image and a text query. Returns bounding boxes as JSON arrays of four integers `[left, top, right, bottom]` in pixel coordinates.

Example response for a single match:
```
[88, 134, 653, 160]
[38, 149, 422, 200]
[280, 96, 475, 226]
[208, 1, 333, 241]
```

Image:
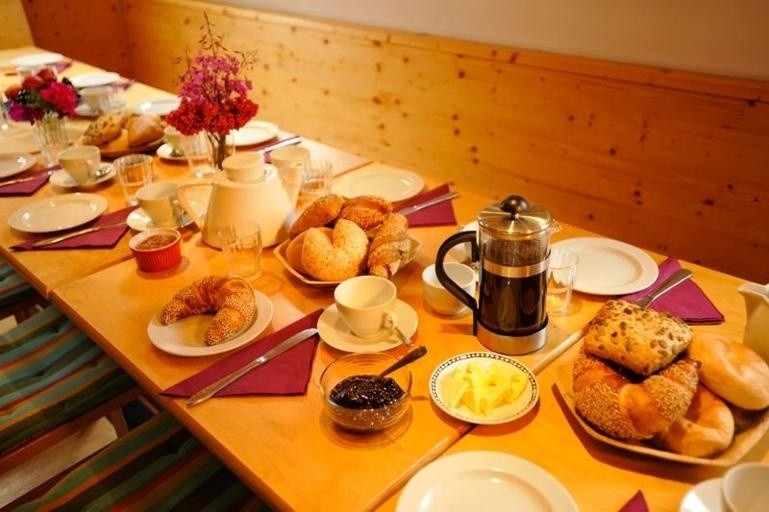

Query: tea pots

[736, 281, 768, 355]
[435, 196, 561, 357]
[176, 151, 304, 252]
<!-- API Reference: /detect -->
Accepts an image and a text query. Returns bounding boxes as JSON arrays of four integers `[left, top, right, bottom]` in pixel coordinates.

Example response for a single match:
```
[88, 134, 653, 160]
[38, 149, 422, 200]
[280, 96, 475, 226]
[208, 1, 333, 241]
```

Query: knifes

[0, 170, 53, 189]
[635, 269, 692, 309]
[31, 219, 126, 248]
[394, 192, 459, 218]
[186, 327, 318, 407]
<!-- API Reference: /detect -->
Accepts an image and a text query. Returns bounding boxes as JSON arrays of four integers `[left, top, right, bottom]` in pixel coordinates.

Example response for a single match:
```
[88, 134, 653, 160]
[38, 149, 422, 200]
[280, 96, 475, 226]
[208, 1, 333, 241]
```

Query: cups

[268, 144, 333, 208]
[460, 219, 479, 261]
[218, 220, 262, 283]
[128, 230, 184, 274]
[133, 177, 186, 224]
[722, 461, 768, 512]
[334, 273, 399, 339]
[163, 124, 185, 155]
[57, 146, 102, 181]
[421, 262, 475, 315]
[74, 72, 127, 117]
[546, 247, 580, 313]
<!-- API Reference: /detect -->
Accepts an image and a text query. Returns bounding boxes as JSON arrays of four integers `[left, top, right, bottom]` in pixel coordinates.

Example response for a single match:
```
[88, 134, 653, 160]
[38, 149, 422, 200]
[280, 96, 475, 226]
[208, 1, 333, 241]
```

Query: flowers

[2, 68, 82, 144]
[160, 11, 261, 173]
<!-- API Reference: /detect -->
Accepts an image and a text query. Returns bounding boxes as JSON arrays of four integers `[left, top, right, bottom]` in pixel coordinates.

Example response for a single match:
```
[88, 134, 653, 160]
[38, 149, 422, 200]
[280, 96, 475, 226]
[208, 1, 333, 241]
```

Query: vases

[28, 111, 71, 164]
[206, 131, 235, 172]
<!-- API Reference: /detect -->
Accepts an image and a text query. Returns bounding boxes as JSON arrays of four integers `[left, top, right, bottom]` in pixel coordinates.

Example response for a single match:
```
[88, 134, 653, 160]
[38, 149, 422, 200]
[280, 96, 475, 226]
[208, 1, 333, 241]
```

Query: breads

[81, 113, 164, 149]
[285, 194, 412, 280]
[573, 299, 769, 457]
[160, 276, 257, 346]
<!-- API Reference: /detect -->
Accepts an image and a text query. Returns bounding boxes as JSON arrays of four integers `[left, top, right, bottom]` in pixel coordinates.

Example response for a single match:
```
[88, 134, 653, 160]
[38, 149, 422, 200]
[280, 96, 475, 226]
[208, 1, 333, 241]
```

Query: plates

[146, 288, 275, 357]
[77, 121, 165, 151]
[156, 142, 213, 162]
[554, 357, 768, 468]
[2, 152, 35, 176]
[317, 297, 419, 354]
[397, 450, 578, 511]
[226, 120, 277, 148]
[330, 166, 425, 207]
[126, 208, 195, 229]
[46, 162, 118, 189]
[429, 352, 540, 426]
[133, 97, 185, 115]
[114, 152, 155, 207]
[273, 232, 422, 287]
[679, 476, 728, 511]
[9, 54, 70, 76]
[7, 190, 111, 234]
[548, 236, 660, 295]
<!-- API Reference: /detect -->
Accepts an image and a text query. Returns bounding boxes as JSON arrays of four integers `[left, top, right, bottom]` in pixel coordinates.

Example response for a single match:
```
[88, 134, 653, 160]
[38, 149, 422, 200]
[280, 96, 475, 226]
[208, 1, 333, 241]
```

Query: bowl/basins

[319, 352, 414, 433]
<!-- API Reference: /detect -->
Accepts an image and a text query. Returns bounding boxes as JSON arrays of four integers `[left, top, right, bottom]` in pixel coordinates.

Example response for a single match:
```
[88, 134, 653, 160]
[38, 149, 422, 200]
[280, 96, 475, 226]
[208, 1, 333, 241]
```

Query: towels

[46, 58, 74, 72]
[157, 306, 325, 398]
[107, 77, 134, 92]
[0, 165, 61, 197]
[9, 203, 142, 251]
[618, 491, 649, 511]
[391, 182, 457, 227]
[621, 254, 726, 326]
